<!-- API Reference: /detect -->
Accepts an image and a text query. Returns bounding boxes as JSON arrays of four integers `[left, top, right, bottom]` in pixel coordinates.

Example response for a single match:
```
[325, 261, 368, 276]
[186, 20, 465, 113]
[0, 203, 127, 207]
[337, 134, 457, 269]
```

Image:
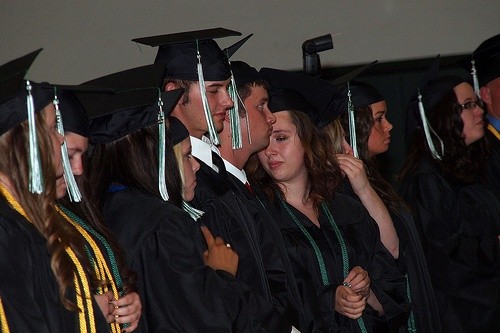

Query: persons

[92, 27, 500, 333]
[0, 47, 144, 333]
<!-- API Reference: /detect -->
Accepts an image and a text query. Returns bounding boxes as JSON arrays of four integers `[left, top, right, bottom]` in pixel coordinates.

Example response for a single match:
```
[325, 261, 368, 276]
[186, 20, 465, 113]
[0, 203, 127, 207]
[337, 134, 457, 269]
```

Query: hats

[0, 27, 500, 222]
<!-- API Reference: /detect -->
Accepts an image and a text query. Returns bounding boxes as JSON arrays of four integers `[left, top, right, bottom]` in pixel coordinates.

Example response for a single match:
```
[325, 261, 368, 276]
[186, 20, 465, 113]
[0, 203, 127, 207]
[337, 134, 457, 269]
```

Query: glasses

[460, 98, 484, 110]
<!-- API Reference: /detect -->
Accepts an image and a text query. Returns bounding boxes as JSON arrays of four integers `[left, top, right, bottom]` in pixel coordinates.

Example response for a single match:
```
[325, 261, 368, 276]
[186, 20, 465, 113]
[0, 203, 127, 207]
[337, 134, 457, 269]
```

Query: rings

[226, 244, 231, 248]
